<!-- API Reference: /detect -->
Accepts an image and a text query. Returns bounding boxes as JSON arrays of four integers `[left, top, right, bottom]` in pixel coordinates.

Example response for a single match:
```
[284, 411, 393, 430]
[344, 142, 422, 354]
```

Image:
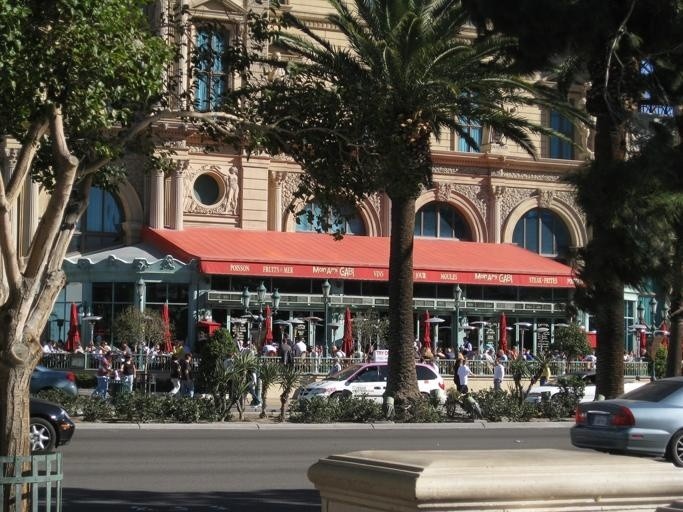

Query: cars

[29, 366, 78, 404]
[28, 397, 75, 454]
[522, 370, 596, 405]
[291, 362, 446, 410]
[569, 377, 683, 468]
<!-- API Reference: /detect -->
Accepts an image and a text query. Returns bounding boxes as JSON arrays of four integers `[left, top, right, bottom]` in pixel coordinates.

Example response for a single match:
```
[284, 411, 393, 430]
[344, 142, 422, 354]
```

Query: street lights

[453, 283, 463, 359]
[321, 276, 333, 358]
[637, 301, 644, 327]
[649, 297, 657, 381]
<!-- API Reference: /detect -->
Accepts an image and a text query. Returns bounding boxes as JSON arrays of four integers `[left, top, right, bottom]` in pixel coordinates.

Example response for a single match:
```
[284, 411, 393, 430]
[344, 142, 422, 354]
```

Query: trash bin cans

[111, 380, 130, 400]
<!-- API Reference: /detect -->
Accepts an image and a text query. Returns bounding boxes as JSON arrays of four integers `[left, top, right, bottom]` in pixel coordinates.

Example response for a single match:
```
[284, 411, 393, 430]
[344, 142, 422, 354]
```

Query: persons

[42, 337, 658, 408]
[222, 167, 239, 215]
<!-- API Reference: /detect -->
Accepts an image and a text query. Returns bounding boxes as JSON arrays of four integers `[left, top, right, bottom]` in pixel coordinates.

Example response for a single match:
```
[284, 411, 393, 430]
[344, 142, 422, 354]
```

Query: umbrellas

[341, 307, 354, 355]
[640, 319, 647, 357]
[423, 309, 435, 348]
[265, 305, 273, 342]
[162, 299, 173, 352]
[499, 311, 510, 353]
[65, 301, 80, 351]
[660, 319, 669, 346]
